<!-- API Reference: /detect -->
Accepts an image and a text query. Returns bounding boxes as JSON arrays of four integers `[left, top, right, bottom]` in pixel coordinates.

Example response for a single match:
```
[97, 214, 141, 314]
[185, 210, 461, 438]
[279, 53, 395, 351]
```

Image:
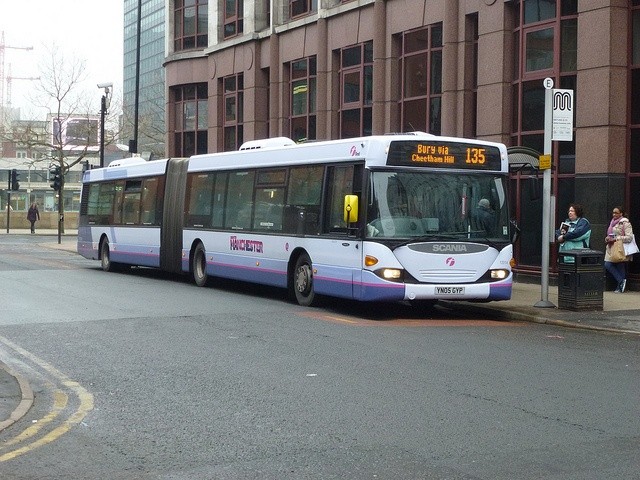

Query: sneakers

[615, 279, 626, 293]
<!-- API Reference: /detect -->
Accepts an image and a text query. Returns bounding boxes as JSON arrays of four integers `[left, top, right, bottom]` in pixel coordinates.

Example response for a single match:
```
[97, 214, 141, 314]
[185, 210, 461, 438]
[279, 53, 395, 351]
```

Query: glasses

[612, 212, 620, 214]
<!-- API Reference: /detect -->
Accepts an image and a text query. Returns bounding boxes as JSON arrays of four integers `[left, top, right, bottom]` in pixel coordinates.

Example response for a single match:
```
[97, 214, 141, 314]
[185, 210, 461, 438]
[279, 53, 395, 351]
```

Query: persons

[470, 198, 491, 236]
[555, 205, 591, 263]
[606, 205, 634, 291]
[27, 201, 39, 232]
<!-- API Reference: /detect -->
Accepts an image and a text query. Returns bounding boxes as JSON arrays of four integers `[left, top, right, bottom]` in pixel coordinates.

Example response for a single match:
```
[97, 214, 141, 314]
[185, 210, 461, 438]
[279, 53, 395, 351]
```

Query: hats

[479, 199, 489, 208]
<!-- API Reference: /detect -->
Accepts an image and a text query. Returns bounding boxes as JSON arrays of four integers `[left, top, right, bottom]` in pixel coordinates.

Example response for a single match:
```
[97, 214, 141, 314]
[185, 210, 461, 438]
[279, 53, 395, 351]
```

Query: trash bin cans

[557, 248, 605, 312]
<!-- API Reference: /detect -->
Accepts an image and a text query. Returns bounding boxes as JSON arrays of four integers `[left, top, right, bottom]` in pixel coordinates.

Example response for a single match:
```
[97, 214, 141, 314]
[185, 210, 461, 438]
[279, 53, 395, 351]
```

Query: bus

[76, 128, 513, 308]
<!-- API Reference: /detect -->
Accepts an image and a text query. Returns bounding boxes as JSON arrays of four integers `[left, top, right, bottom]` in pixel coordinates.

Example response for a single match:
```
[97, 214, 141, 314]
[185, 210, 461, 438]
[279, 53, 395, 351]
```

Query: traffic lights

[6, 168, 19, 192]
[49, 169, 65, 192]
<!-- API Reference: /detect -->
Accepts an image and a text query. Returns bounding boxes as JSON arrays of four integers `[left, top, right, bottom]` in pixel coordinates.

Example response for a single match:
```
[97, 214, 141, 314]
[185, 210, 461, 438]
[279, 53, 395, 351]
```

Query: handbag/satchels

[622, 222, 639, 256]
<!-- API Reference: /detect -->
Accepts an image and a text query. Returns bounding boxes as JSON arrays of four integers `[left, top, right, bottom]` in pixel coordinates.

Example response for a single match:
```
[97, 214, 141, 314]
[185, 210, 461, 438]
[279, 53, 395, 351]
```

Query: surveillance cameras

[96, 82, 114, 91]
